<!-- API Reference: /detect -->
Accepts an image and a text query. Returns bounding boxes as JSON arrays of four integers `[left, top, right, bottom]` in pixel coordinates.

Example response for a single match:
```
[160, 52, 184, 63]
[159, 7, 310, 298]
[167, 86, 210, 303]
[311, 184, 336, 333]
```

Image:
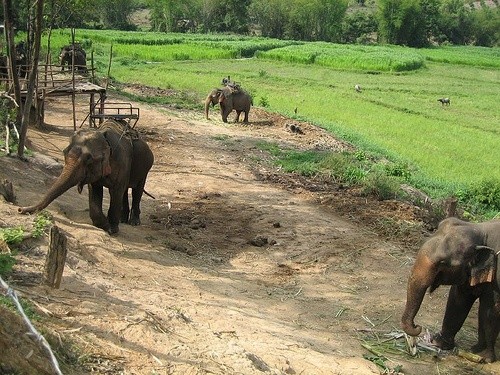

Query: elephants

[402, 216, 500, 364]
[59, 45, 87, 72]
[16, 118, 157, 237]
[204, 86, 254, 124]
[437, 97, 450, 105]
